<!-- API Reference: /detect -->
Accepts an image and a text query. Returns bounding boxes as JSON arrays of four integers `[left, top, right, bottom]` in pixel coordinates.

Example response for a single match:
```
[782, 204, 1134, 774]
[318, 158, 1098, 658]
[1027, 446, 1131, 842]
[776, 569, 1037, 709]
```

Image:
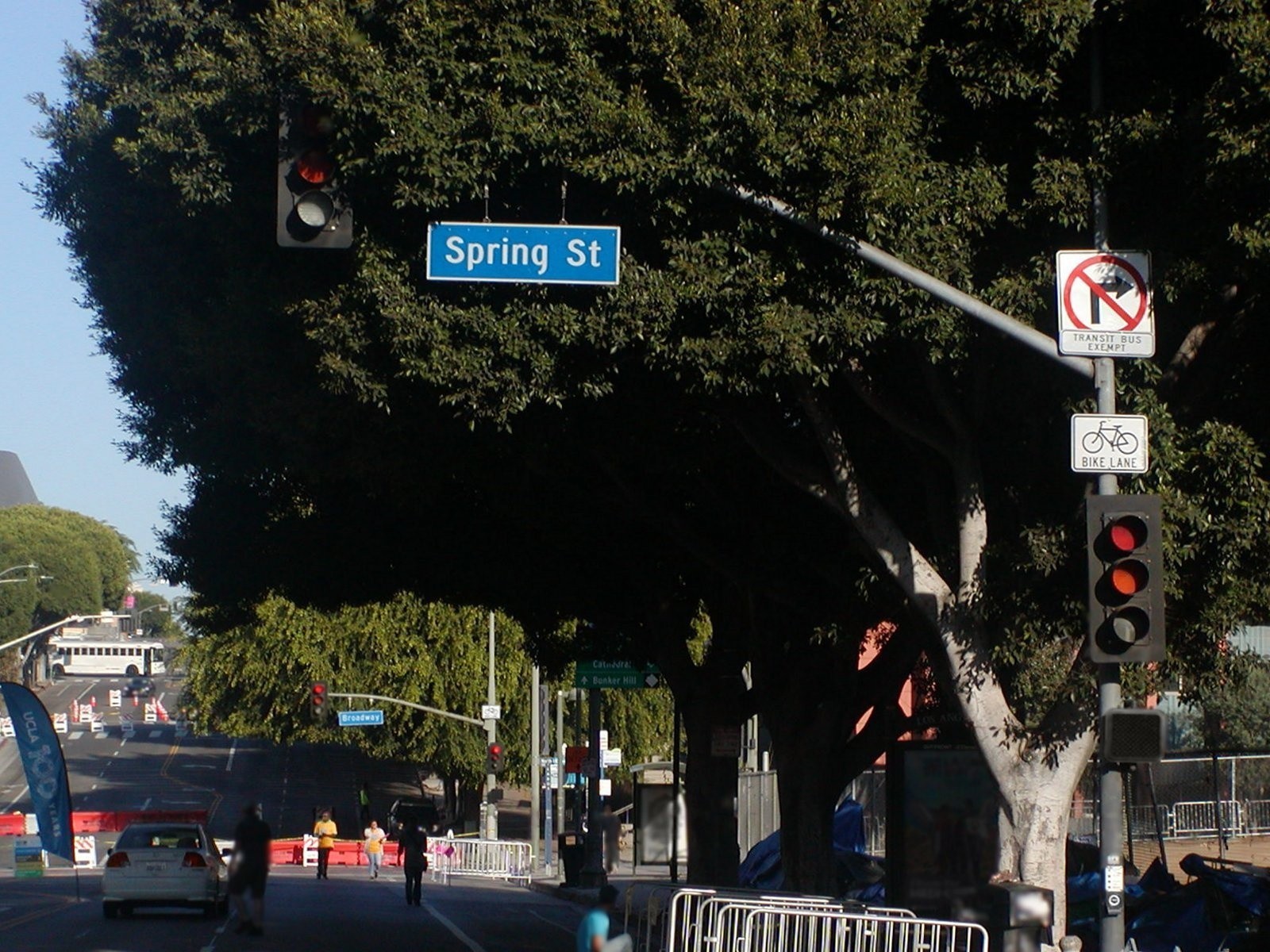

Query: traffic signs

[574, 651, 661, 689]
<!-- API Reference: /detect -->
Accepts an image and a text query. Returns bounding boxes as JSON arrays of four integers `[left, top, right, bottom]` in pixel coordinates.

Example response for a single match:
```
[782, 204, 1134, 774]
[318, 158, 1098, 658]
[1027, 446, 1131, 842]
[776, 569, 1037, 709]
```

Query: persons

[314, 810, 338, 880]
[602, 805, 621, 875]
[228, 802, 273, 937]
[577, 885, 633, 952]
[397, 819, 427, 907]
[364, 819, 387, 880]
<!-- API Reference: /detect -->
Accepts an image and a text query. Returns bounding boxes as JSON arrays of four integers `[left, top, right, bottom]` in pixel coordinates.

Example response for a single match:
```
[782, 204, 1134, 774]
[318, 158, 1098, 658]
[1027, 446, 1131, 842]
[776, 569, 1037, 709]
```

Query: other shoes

[234, 918, 252, 935]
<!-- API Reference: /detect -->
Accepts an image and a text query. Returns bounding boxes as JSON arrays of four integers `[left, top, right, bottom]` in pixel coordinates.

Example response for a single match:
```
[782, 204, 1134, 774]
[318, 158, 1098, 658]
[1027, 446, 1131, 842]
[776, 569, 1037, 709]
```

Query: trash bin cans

[981, 882, 1055, 952]
[559, 831, 585, 887]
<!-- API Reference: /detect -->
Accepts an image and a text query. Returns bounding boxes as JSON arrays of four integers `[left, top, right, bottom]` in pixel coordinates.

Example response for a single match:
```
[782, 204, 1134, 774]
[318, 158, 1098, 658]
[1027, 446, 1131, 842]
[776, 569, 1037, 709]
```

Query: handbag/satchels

[423, 857, 427, 871]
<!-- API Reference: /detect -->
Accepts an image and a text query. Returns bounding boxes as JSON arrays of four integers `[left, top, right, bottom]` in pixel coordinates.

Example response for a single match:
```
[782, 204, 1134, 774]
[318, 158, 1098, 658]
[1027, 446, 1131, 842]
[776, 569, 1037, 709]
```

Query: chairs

[132, 836, 149, 847]
[177, 838, 197, 848]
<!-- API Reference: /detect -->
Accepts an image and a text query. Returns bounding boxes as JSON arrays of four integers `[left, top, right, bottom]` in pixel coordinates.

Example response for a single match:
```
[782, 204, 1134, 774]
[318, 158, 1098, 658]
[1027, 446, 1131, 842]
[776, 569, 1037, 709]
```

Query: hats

[242, 800, 256, 811]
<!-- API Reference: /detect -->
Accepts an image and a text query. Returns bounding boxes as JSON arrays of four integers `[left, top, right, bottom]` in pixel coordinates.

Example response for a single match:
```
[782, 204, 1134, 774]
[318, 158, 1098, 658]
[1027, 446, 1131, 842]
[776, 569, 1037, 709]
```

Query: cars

[121, 677, 155, 698]
[100, 820, 233, 921]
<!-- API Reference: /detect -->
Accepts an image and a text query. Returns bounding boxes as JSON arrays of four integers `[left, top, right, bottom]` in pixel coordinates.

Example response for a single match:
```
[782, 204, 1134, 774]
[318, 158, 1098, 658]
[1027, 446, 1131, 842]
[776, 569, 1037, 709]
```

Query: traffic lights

[1102, 706, 1167, 763]
[277, 111, 354, 248]
[1087, 494, 1167, 662]
[310, 680, 328, 720]
[485, 741, 504, 774]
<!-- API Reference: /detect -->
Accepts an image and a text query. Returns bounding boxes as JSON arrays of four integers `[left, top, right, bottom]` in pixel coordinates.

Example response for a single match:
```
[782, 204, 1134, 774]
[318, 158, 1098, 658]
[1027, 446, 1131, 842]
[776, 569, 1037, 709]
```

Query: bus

[20, 634, 167, 680]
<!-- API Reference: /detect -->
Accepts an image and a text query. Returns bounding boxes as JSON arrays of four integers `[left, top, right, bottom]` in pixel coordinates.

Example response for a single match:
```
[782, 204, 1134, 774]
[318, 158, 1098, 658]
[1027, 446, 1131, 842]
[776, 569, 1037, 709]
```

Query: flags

[0, 682, 75, 863]
